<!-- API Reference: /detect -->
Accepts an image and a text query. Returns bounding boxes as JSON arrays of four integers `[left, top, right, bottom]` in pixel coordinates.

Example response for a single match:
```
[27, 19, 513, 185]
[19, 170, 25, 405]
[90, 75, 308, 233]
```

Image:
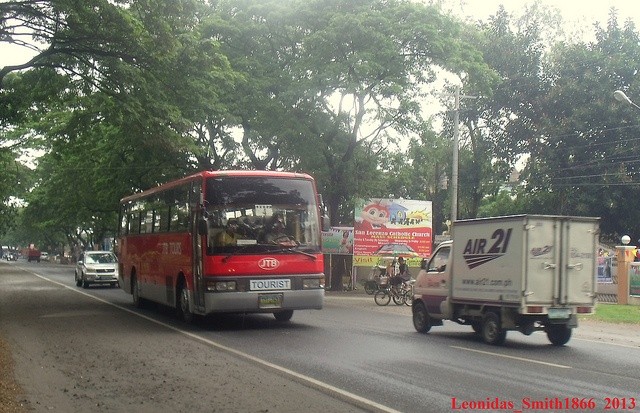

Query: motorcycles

[364, 262, 391, 294]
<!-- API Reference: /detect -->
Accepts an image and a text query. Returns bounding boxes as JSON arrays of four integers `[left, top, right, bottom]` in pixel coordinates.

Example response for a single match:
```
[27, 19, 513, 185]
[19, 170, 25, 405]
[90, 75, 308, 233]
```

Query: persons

[338, 232, 353, 254]
[264, 210, 300, 245]
[216, 217, 241, 248]
[391, 256, 411, 286]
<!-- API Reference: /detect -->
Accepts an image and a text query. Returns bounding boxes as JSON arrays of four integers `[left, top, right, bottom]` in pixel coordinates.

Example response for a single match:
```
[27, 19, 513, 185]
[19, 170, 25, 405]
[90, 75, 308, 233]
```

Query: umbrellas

[372, 242, 416, 263]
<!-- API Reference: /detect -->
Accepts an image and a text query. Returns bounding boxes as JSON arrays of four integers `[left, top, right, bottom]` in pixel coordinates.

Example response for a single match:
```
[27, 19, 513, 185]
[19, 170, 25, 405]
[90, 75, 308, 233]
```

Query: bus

[117, 169, 330, 330]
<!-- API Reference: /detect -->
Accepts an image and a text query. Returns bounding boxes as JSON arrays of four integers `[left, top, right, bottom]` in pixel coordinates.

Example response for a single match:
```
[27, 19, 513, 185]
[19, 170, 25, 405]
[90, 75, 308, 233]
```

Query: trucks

[412, 213, 601, 344]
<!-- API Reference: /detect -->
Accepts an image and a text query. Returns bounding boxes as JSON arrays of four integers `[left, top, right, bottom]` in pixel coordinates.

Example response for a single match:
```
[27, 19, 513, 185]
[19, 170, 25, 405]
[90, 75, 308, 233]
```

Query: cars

[75, 250, 119, 287]
[3, 246, 50, 263]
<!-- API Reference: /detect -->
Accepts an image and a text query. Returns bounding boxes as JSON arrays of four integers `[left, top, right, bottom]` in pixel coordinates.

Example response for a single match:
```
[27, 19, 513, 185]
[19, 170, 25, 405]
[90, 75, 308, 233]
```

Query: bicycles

[374, 281, 407, 308]
[403, 289, 413, 307]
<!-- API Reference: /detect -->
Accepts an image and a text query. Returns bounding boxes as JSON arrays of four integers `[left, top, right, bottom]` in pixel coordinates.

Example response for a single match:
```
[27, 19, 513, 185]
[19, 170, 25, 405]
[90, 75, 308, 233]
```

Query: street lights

[611, 89, 640, 110]
